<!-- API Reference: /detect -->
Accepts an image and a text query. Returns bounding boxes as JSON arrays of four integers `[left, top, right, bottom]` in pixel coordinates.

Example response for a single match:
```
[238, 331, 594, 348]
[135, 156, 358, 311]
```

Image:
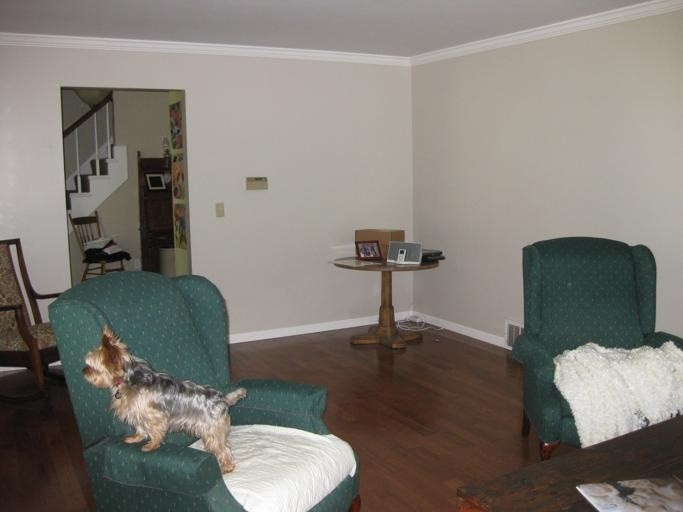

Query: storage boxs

[355, 228, 404, 256]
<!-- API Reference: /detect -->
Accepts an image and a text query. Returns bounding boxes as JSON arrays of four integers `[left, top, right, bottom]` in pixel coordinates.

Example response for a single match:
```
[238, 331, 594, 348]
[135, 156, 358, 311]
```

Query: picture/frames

[355, 240, 382, 259]
[143, 172, 166, 191]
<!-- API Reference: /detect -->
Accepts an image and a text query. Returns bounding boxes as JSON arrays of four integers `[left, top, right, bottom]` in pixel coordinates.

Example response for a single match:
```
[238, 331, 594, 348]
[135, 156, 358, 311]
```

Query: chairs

[2, 237, 68, 422]
[68, 211, 127, 283]
[512, 236, 683, 461]
[48, 269, 359, 511]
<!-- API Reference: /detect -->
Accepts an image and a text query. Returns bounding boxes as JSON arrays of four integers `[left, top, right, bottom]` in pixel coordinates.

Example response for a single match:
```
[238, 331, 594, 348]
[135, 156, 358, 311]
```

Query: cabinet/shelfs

[136, 149, 175, 273]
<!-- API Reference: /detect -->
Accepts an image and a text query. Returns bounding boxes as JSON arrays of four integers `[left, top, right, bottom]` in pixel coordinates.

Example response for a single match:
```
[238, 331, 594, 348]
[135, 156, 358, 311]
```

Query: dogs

[82, 324, 248, 476]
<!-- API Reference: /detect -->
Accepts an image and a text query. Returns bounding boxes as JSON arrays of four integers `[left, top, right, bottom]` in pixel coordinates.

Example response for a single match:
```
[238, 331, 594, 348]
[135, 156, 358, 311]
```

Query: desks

[333, 256, 439, 349]
[456, 413, 683, 512]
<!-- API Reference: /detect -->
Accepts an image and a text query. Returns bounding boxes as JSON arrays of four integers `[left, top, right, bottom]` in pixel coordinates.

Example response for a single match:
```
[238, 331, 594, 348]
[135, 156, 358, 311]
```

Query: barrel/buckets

[159, 247, 176, 277]
